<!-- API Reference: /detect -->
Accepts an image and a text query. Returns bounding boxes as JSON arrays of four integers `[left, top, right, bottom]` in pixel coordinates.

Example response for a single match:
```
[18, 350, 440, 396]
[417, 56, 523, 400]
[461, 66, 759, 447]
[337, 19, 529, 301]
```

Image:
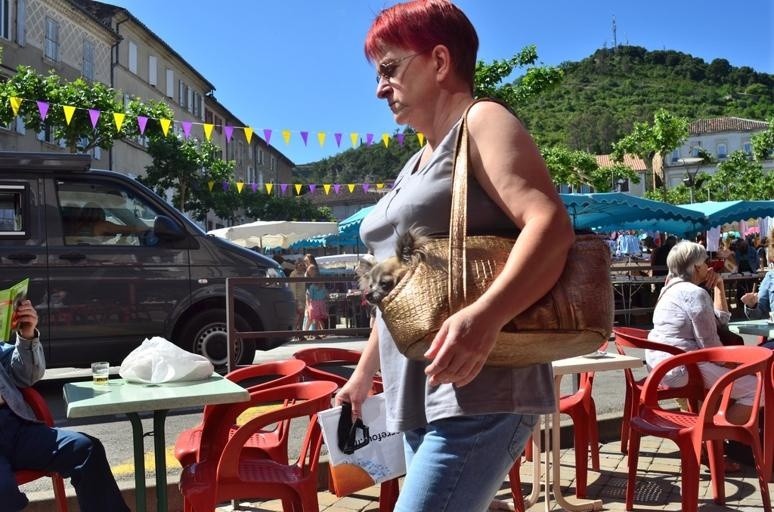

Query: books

[0, 279, 31, 345]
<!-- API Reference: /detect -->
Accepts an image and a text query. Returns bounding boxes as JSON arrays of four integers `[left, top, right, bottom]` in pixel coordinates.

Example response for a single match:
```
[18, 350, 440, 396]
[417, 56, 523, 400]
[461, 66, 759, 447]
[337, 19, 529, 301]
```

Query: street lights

[676, 157, 704, 203]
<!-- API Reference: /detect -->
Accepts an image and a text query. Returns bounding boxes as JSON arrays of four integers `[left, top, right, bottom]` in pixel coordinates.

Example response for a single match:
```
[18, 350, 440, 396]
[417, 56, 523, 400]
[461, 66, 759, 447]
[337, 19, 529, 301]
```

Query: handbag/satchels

[377, 234, 614, 368]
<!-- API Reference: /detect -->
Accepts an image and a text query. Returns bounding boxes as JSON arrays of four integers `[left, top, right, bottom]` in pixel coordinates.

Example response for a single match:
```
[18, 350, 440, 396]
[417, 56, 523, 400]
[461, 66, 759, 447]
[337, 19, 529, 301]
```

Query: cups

[90, 359, 110, 386]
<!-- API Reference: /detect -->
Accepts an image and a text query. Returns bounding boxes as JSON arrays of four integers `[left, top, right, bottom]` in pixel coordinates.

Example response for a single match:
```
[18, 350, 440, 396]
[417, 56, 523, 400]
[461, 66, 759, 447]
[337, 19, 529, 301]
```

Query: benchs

[64, 202, 113, 236]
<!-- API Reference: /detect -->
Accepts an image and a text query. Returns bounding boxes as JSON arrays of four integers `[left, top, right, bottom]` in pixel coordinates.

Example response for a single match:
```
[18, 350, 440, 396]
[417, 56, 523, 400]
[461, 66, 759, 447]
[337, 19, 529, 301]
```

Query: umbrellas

[556, 190, 705, 237]
[206, 219, 339, 255]
[600, 199, 773, 234]
[290, 204, 374, 287]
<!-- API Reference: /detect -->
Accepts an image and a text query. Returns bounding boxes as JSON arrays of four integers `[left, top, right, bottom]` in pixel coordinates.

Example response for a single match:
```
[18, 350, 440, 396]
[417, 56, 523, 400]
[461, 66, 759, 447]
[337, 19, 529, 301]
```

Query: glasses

[376, 50, 427, 82]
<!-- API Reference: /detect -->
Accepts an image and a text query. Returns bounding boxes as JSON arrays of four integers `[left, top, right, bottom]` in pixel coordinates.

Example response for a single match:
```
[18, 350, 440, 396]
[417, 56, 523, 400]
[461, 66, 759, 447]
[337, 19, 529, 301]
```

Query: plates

[583, 352, 607, 358]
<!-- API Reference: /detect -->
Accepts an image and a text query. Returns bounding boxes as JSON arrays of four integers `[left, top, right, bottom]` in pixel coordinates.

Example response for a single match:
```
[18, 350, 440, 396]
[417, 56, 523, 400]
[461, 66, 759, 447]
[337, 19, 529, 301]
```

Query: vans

[0, 147, 302, 379]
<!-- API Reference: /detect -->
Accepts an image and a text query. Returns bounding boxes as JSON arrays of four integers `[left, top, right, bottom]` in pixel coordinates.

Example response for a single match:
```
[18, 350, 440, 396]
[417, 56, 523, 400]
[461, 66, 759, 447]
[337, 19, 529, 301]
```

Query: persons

[643, 237, 764, 473]
[647, 223, 772, 294]
[272, 251, 329, 343]
[333, 1, 574, 511]
[68, 202, 146, 248]
[0, 298, 131, 511]
[740, 237, 773, 349]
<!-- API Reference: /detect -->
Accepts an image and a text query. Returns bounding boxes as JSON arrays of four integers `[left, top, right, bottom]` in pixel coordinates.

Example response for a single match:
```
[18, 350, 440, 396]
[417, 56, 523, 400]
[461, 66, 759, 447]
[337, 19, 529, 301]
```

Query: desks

[609, 260, 766, 325]
